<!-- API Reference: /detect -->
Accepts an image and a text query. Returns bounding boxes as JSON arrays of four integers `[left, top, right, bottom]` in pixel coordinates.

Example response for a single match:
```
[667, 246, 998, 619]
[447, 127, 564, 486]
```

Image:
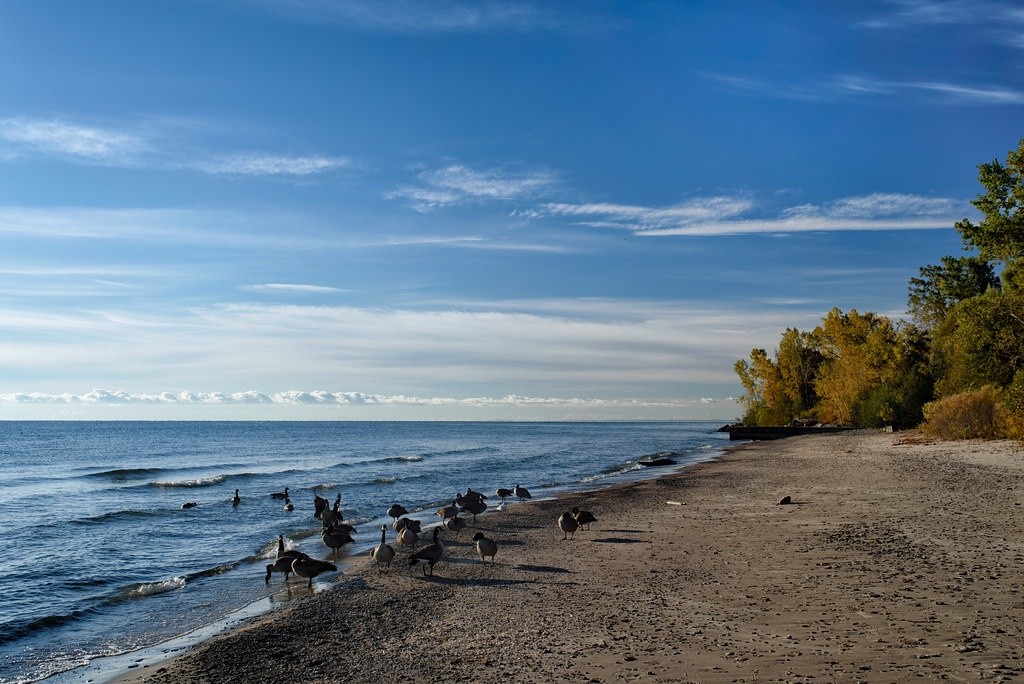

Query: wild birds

[448, 516, 466, 535]
[265, 535, 338, 590]
[496, 488, 514, 502]
[313, 493, 357, 562]
[393, 517, 421, 552]
[369, 524, 396, 574]
[283, 499, 295, 511]
[405, 525, 445, 577]
[231, 488, 241, 506]
[270, 487, 290, 499]
[435, 500, 460, 526]
[473, 532, 498, 567]
[455, 488, 488, 523]
[516, 484, 532, 501]
[573, 506, 598, 531]
[387, 504, 409, 523]
[558, 512, 580, 540]
